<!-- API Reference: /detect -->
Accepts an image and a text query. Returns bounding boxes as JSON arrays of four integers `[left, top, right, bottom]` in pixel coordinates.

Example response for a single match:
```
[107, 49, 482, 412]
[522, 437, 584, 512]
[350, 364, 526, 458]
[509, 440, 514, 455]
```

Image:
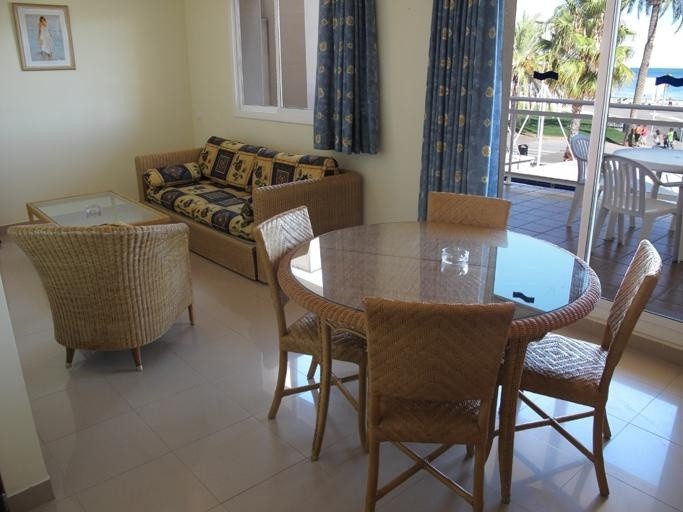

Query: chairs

[254, 205, 371, 454]
[7, 224, 195, 372]
[485, 239, 663, 498]
[568, 133, 635, 245]
[591, 153, 683, 263]
[426, 191, 511, 229]
[363, 296, 516, 512]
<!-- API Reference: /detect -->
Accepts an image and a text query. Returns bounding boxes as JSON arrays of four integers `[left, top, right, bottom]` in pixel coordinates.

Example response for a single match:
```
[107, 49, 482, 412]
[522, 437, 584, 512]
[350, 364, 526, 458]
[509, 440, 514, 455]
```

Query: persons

[641, 125, 648, 147]
[635, 125, 641, 144]
[38, 16, 55, 58]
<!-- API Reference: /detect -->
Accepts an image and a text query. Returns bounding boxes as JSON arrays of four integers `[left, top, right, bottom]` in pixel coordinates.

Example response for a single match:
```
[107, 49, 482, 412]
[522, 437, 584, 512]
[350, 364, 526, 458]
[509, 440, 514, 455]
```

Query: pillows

[240, 198, 253, 221]
[143, 162, 201, 188]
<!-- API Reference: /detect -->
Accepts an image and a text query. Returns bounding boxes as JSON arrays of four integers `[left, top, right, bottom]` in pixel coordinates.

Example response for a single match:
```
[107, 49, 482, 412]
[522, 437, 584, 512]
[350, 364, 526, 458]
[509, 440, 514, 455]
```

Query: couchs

[135, 134, 364, 285]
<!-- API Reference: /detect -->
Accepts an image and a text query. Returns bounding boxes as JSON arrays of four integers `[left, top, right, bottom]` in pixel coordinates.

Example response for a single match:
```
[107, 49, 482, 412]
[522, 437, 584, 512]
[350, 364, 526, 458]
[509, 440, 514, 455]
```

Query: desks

[276, 221, 602, 504]
[613, 147, 683, 264]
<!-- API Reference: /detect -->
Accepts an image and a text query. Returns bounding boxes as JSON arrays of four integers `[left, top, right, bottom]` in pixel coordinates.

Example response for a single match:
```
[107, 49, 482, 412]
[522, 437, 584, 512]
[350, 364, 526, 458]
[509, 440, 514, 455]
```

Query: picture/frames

[12, 3, 77, 71]
